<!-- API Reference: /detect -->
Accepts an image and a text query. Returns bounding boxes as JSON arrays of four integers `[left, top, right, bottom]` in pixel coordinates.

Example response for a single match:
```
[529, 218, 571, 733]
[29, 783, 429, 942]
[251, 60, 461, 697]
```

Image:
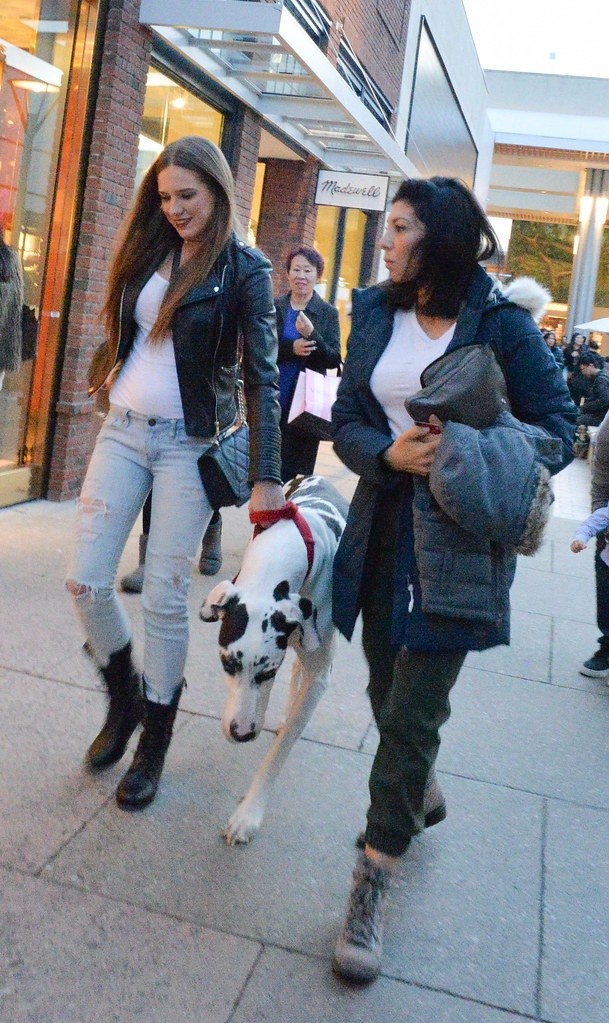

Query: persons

[540, 327, 609, 462]
[118, 486, 226, 595]
[0, 230, 25, 394]
[327, 173, 583, 984]
[569, 409, 609, 679]
[64, 135, 286, 810]
[271, 244, 344, 483]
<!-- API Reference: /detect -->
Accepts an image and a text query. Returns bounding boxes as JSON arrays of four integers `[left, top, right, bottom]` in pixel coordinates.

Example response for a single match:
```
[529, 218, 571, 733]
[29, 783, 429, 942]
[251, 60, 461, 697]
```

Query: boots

[86, 638, 144, 770]
[332, 852, 394, 980]
[116, 674, 185, 810]
[121, 535, 149, 593]
[357, 762, 448, 850]
[199, 511, 223, 575]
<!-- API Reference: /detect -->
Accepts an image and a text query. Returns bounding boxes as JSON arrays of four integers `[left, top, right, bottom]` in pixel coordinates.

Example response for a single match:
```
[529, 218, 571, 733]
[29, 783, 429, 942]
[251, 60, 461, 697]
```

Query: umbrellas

[573, 318, 609, 332]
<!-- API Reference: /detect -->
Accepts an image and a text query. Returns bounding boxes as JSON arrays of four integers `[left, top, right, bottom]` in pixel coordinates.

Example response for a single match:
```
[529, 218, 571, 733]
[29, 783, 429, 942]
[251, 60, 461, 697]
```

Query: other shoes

[579, 637, 609, 677]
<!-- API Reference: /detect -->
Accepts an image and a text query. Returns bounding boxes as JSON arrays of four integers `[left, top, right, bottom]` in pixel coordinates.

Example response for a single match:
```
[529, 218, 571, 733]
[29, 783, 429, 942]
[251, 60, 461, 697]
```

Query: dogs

[199, 477, 352, 850]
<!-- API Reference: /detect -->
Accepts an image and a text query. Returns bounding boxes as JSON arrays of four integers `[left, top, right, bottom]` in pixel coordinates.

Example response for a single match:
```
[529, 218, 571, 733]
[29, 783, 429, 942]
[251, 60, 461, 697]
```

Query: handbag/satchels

[405, 342, 510, 430]
[287, 360, 345, 442]
[196, 423, 254, 511]
